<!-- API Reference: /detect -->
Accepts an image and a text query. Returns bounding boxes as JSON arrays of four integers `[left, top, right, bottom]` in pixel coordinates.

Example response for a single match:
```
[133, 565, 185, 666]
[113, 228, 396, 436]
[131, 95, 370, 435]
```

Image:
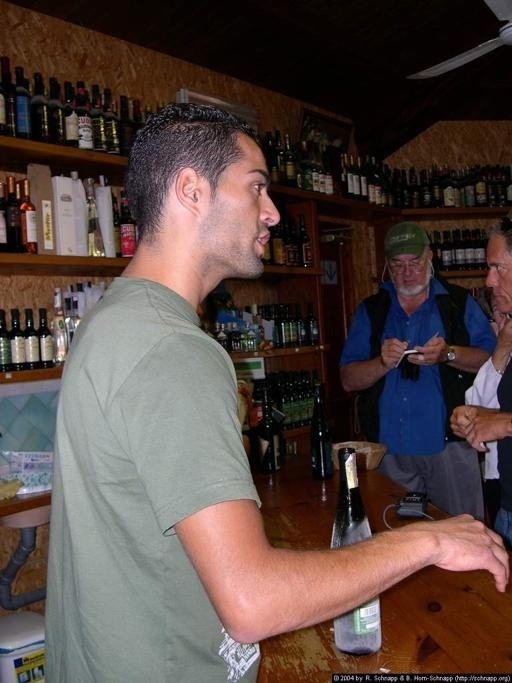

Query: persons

[43, 99, 511, 683]
[464, 285, 512, 524]
[450, 220, 512, 545]
[338, 219, 496, 526]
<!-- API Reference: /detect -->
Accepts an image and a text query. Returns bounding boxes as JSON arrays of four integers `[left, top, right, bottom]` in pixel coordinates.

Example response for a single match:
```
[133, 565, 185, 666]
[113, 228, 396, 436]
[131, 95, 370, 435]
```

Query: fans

[408, 0, 512, 79]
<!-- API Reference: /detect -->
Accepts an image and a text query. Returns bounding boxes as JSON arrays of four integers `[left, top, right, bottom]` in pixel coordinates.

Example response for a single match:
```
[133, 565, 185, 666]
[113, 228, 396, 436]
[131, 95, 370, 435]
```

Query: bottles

[255, 367, 323, 430]
[312, 383, 335, 479]
[251, 128, 315, 269]
[251, 387, 289, 476]
[319, 144, 510, 208]
[0, 279, 103, 372]
[0, 66, 147, 258]
[202, 300, 320, 352]
[330, 447, 386, 656]
[426, 227, 486, 270]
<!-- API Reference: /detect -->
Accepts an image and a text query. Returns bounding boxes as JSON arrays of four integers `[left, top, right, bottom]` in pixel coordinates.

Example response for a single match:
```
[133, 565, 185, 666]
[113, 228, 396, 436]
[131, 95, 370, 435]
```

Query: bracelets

[497, 368, 506, 375]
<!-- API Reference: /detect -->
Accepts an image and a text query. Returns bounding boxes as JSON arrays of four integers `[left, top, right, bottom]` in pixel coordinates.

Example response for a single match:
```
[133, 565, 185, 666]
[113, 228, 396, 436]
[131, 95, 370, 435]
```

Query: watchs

[447, 344, 456, 363]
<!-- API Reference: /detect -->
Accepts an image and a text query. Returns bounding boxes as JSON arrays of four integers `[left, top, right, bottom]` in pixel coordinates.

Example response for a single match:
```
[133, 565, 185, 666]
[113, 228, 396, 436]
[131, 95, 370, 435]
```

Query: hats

[384, 222, 430, 259]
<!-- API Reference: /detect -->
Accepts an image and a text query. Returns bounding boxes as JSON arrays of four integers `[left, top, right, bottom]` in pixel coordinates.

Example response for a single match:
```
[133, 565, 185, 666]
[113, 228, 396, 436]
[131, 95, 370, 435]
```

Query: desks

[256, 432, 512, 681]
